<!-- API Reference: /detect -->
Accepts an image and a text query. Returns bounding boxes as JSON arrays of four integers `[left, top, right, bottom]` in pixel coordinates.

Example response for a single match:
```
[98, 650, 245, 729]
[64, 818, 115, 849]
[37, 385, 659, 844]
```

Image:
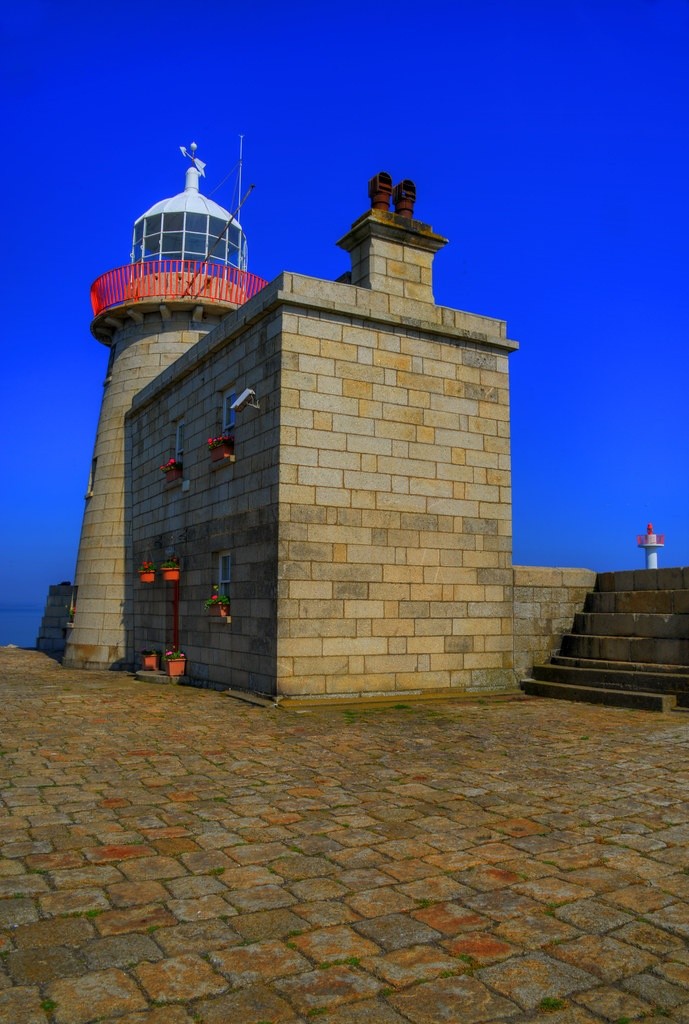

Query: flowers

[138, 645, 160, 654]
[138, 561, 156, 571]
[203, 595, 229, 611]
[160, 458, 182, 472]
[161, 645, 185, 661]
[207, 434, 234, 450]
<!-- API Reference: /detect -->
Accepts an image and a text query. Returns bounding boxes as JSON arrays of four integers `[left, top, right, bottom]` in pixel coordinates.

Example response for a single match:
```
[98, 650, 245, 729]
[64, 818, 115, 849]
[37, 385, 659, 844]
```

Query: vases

[209, 444, 232, 462]
[166, 470, 181, 482]
[165, 658, 186, 676]
[137, 570, 154, 583]
[142, 654, 159, 670]
[208, 602, 229, 617]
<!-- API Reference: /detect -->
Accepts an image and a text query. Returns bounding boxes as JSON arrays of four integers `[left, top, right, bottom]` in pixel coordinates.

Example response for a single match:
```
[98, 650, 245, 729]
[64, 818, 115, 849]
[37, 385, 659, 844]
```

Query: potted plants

[160, 561, 180, 580]
